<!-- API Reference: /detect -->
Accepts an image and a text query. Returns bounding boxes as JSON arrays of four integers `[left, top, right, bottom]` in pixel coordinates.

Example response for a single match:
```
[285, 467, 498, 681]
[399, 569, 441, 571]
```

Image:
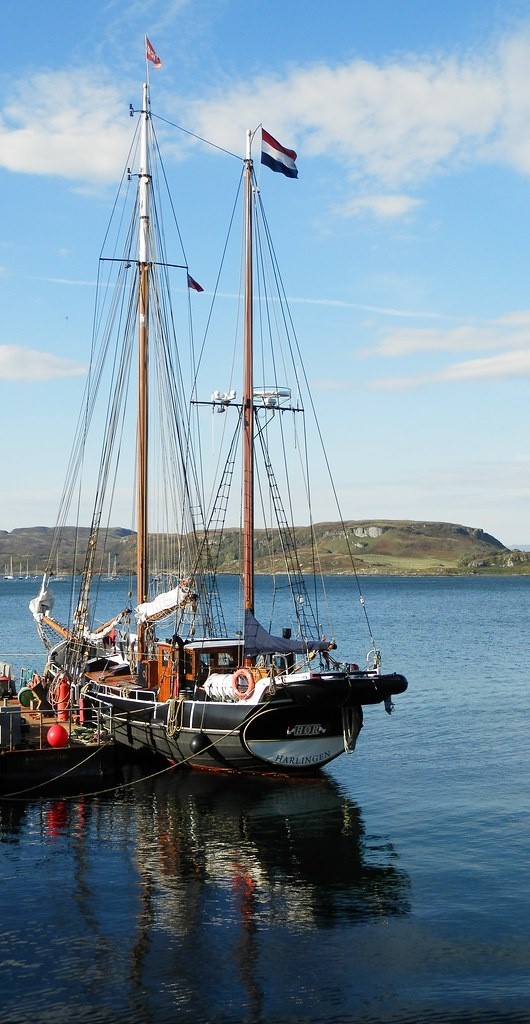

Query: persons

[28, 669, 41, 710]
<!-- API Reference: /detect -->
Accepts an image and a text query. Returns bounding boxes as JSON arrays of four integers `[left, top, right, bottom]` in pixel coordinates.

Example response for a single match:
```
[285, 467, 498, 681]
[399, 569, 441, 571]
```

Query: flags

[260, 129, 299, 179]
[186, 275, 202, 292]
[147, 39, 161, 70]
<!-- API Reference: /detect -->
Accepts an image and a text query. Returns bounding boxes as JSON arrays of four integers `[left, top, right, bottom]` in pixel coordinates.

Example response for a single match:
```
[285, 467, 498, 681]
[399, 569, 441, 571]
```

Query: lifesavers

[231, 668, 255, 700]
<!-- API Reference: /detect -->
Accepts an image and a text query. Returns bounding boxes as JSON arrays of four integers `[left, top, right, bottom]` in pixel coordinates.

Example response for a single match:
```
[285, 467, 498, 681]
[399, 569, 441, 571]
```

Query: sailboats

[4, 553, 122, 581]
[28, 34, 407, 778]
